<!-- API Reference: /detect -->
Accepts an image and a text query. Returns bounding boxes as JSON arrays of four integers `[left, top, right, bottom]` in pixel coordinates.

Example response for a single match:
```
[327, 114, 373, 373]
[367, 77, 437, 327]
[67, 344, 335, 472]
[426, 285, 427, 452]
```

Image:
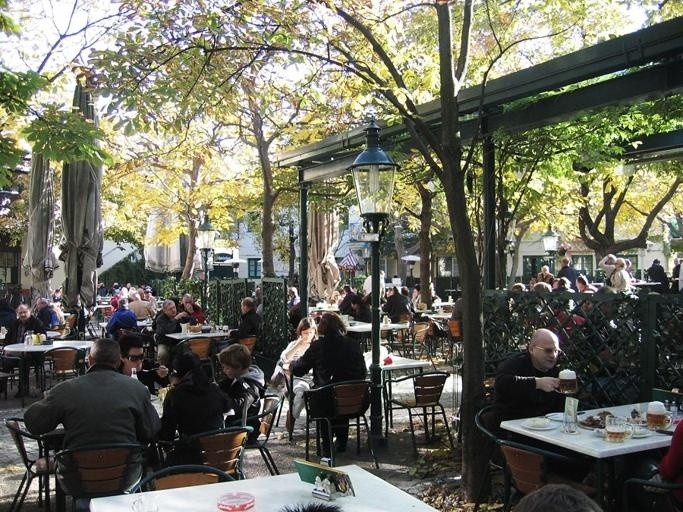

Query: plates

[632, 430, 651, 438]
[521, 417, 559, 430]
[625, 421, 647, 427]
[666, 424, 677, 432]
[546, 411, 564, 421]
[578, 423, 595, 430]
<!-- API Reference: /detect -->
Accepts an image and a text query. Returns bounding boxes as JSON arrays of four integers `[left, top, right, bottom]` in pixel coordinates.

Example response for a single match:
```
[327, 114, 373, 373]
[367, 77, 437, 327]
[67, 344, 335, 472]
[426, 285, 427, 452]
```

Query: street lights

[194, 211, 218, 314]
[223, 247, 246, 279]
[342, 119, 401, 453]
[541, 221, 559, 275]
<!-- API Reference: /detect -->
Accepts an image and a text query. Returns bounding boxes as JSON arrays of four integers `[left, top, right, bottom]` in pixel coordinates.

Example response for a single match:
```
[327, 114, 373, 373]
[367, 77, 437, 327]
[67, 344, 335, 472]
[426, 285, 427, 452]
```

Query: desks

[499, 401, 683, 510]
[90, 464, 439, 510]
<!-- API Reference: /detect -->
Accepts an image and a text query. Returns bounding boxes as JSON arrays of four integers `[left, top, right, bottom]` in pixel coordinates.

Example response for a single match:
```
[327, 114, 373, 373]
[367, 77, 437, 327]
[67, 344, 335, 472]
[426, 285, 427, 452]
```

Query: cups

[133, 492, 160, 512]
[554, 372, 577, 394]
[0, 326, 7, 335]
[31, 334, 45, 345]
[606, 415, 634, 442]
[208, 321, 228, 334]
[157, 392, 165, 415]
[382, 315, 391, 325]
[216, 490, 256, 512]
[182, 324, 186, 335]
[23, 331, 33, 347]
[563, 408, 577, 434]
[646, 409, 672, 432]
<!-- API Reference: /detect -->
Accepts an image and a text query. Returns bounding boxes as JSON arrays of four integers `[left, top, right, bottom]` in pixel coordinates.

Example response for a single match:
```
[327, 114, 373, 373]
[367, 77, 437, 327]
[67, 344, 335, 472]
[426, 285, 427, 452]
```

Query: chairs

[132, 465, 236, 495]
[475, 404, 512, 507]
[619, 477, 682, 511]
[53, 443, 144, 512]
[1, 298, 462, 407]
[158, 426, 254, 480]
[275, 353, 455, 469]
[5, 416, 62, 511]
[227, 394, 284, 475]
[497, 439, 597, 510]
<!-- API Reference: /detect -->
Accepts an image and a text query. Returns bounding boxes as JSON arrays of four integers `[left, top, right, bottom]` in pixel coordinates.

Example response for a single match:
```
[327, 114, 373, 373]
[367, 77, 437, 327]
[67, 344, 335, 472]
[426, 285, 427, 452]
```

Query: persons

[0, 281, 442, 512]
[450, 254, 683, 512]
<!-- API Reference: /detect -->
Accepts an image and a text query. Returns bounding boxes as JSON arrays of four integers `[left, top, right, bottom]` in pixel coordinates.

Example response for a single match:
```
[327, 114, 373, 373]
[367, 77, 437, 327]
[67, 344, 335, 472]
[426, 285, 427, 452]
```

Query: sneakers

[287, 411, 295, 433]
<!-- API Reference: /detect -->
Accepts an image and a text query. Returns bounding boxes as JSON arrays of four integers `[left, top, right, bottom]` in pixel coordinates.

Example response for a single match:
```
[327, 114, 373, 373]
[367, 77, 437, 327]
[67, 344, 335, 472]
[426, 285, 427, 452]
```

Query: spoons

[142, 367, 165, 372]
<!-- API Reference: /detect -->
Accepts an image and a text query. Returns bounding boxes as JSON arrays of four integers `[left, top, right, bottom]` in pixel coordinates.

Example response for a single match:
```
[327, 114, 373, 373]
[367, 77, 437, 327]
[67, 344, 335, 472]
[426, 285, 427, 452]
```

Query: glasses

[124, 353, 144, 361]
[535, 344, 559, 354]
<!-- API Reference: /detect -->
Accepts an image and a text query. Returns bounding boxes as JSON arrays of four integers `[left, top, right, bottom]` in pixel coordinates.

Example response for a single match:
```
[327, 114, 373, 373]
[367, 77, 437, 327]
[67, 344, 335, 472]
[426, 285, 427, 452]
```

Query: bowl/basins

[188, 325, 201, 333]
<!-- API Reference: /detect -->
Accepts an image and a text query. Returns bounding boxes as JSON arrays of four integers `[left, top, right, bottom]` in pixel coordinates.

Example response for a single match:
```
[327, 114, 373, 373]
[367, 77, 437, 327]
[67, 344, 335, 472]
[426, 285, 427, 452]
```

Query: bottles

[664, 399, 677, 414]
[130, 367, 137, 380]
